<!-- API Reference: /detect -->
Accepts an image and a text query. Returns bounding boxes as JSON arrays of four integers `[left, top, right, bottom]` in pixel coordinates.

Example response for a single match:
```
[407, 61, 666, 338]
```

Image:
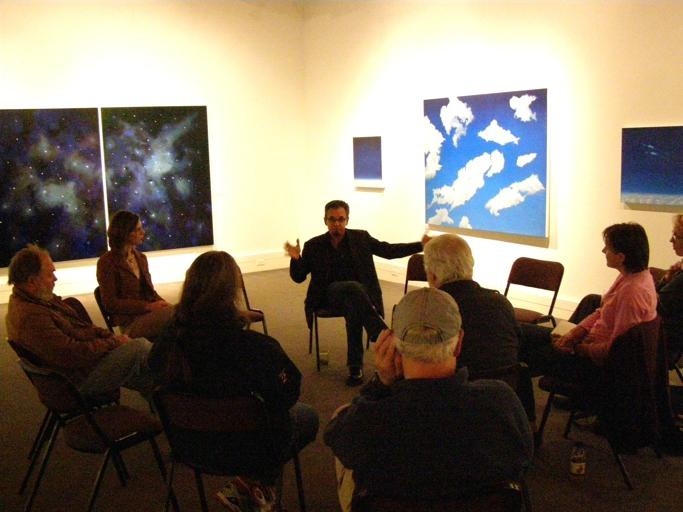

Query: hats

[393, 288, 462, 345]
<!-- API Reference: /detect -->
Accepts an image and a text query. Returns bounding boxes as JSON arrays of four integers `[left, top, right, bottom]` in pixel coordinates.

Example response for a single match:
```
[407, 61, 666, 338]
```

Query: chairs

[92, 286, 120, 335]
[309, 309, 370, 372]
[18, 359, 179, 511]
[535, 313, 663, 490]
[5, 296, 121, 460]
[404, 254, 428, 295]
[504, 257, 564, 328]
[151, 391, 320, 512]
[352, 479, 531, 512]
[235, 265, 269, 337]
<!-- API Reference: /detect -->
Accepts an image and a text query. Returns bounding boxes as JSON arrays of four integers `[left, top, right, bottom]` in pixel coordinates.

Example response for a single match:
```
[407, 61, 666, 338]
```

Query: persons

[657, 213, 682, 361]
[95, 210, 178, 343]
[323, 289, 536, 511]
[282, 198, 431, 384]
[515, 223, 657, 374]
[421, 234, 523, 398]
[146, 250, 319, 511]
[6, 246, 155, 405]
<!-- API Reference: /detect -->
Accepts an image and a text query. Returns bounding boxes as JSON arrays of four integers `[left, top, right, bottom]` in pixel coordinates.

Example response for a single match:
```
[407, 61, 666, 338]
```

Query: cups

[319, 349, 329, 366]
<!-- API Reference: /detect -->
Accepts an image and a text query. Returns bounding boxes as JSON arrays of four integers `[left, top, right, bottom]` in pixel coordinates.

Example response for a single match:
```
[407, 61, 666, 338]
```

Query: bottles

[567, 443, 586, 482]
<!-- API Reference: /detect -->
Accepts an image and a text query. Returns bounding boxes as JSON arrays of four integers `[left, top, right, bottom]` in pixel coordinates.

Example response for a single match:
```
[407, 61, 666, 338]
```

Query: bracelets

[570, 343, 577, 356]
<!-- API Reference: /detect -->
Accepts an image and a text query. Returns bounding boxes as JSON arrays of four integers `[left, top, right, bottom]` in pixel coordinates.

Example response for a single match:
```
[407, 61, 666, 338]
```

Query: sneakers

[218, 477, 279, 512]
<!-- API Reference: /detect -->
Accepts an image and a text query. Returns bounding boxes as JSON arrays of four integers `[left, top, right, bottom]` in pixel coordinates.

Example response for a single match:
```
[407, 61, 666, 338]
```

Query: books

[550, 319, 576, 335]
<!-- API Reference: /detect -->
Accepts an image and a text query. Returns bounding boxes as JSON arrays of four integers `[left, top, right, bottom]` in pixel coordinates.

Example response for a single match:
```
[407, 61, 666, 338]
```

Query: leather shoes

[348, 364, 364, 385]
